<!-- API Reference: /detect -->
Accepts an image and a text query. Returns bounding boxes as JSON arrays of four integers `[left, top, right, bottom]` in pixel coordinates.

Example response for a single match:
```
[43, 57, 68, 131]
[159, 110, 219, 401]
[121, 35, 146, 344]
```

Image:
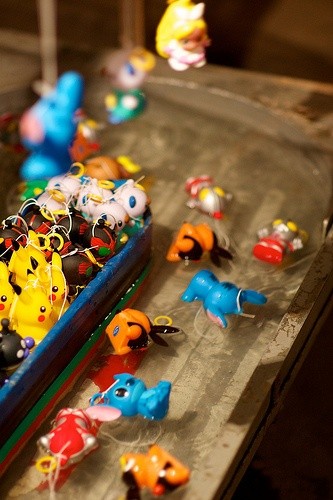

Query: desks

[0, 58, 333, 500]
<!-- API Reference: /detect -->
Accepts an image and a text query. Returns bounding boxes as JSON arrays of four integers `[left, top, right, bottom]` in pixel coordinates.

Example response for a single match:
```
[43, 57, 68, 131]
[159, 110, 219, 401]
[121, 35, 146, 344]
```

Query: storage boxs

[0, 206, 155, 476]
